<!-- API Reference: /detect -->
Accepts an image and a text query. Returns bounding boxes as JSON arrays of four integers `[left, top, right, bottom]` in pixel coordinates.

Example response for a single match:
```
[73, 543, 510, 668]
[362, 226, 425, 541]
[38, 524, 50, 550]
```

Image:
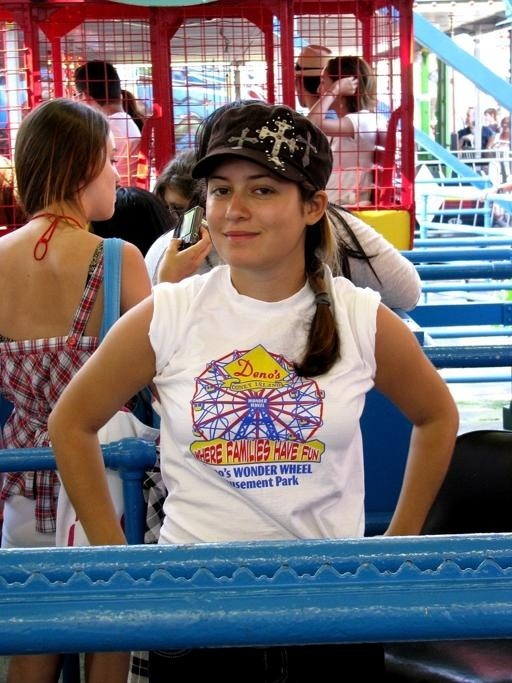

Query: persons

[0, 44, 512, 311]
[47, 100, 459, 683]
[0, 98, 214, 683]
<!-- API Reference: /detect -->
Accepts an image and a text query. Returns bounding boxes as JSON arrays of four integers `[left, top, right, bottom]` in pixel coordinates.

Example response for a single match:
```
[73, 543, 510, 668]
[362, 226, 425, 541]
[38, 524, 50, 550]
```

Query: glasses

[168, 208, 186, 220]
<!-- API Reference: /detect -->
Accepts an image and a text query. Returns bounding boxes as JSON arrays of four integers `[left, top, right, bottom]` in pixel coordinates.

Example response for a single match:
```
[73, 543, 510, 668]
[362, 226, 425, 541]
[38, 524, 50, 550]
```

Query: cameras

[177, 206, 204, 243]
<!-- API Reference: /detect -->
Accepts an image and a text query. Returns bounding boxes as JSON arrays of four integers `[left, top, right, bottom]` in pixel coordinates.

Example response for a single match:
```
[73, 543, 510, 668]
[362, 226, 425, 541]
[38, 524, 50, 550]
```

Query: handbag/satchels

[54, 238, 159, 546]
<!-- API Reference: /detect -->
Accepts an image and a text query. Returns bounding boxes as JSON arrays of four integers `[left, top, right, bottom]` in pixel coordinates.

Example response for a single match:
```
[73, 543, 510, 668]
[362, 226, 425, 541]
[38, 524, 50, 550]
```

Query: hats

[192, 104, 333, 191]
[295, 45, 332, 77]
